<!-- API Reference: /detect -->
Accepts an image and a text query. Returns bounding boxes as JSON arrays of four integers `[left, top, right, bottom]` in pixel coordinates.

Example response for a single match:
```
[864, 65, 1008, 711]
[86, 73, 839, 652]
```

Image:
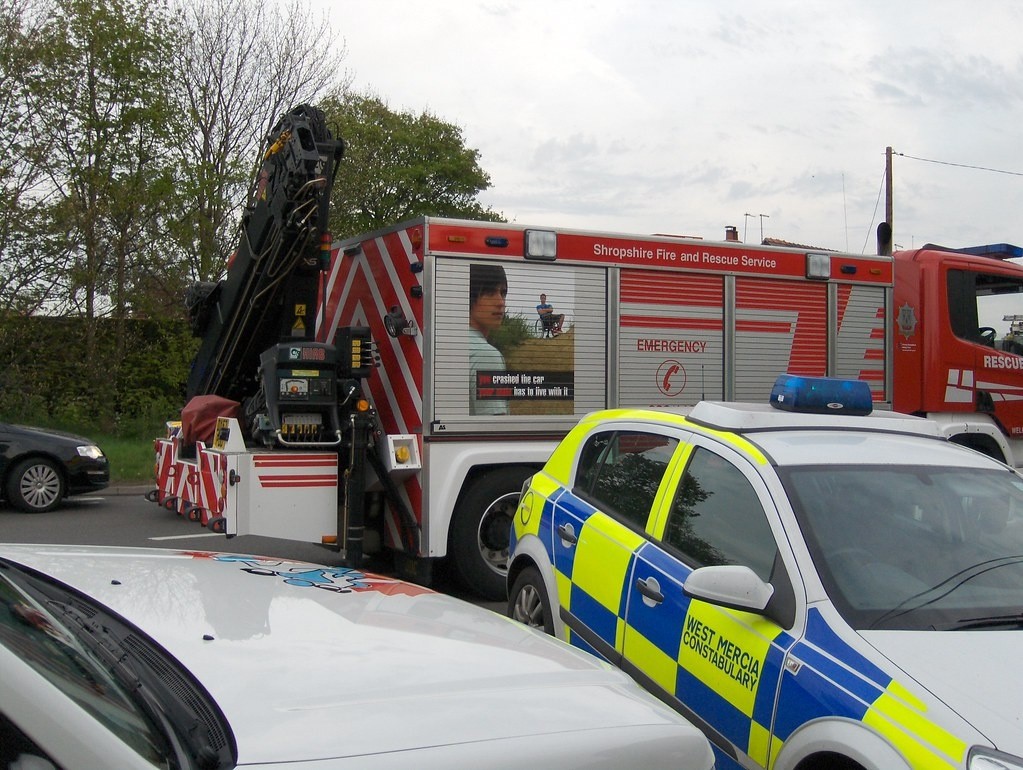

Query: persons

[535, 293, 565, 336]
[468, 264, 509, 415]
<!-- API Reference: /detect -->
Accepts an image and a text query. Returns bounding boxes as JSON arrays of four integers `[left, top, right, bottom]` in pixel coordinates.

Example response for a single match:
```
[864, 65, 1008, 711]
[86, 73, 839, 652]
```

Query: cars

[497, 373, 1022, 770]
[0, 536, 718, 770]
[0, 415, 113, 515]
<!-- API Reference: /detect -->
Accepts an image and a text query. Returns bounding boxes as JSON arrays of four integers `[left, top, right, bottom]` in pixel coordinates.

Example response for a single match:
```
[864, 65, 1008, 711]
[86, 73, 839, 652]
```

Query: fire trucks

[143, 101, 1022, 605]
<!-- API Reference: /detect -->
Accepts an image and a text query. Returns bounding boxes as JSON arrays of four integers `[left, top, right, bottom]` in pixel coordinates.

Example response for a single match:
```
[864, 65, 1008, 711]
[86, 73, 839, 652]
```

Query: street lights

[759, 214, 770, 243]
[744, 212, 756, 244]
